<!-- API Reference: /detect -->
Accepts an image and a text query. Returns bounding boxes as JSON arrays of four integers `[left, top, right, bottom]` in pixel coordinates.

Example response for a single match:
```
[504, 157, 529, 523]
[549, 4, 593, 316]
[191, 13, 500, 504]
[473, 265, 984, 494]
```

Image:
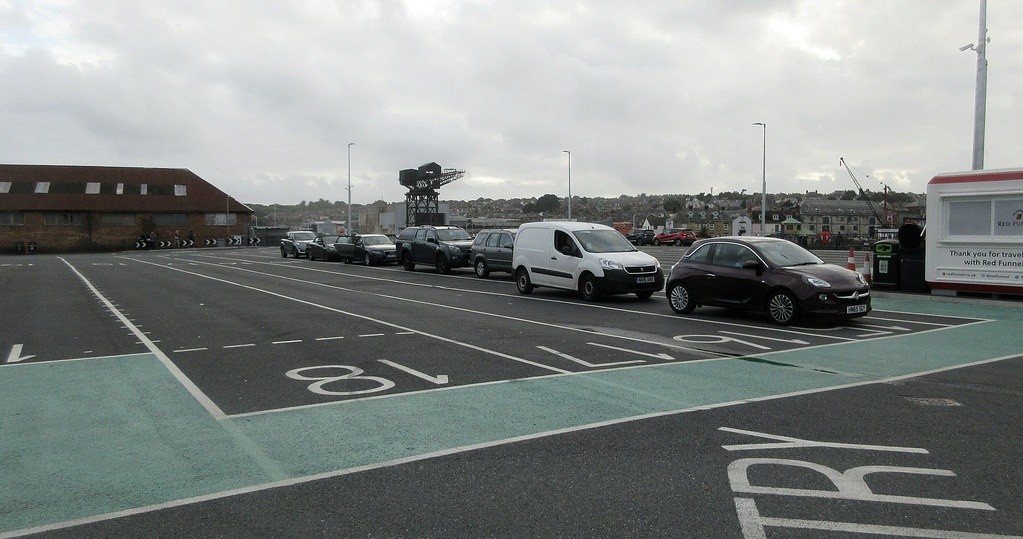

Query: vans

[511, 220, 664, 299]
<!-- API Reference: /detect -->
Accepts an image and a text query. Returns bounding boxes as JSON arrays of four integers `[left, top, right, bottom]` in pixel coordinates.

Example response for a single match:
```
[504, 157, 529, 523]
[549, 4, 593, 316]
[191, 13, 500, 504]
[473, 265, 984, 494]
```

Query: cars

[306, 235, 342, 261]
[334, 234, 402, 265]
[665, 236, 872, 327]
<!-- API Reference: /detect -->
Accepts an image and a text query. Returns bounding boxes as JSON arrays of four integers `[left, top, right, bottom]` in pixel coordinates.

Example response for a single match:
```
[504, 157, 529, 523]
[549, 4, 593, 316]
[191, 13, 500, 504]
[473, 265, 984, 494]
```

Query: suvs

[625, 229, 654, 244]
[652, 228, 696, 246]
[469, 229, 519, 278]
[280, 231, 321, 258]
[396, 225, 475, 273]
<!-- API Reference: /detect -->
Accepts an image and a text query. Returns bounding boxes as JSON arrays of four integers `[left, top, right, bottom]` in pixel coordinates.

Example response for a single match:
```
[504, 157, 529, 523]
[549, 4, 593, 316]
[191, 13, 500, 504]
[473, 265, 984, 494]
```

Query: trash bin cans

[28, 240, 36, 254]
[16, 241, 24, 254]
[872, 239, 899, 289]
[898, 221, 925, 291]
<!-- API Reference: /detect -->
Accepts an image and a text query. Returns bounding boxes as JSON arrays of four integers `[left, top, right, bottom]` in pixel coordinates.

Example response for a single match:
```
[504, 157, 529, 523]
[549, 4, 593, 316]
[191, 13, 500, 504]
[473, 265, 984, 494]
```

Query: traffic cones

[861, 254, 872, 284]
[846, 247, 856, 271]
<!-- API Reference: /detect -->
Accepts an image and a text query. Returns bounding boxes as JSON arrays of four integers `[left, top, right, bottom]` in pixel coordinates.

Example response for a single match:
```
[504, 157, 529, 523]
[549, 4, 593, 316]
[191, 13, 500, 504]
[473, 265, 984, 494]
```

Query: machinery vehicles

[840, 156, 903, 239]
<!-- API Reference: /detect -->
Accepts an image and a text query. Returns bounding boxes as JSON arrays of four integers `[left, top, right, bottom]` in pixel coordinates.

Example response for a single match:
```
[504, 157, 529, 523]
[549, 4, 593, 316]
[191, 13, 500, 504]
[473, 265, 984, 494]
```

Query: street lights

[562, 150, 570, 219]
[348, 142, 355, 236]
[227, 194, 231, 240]
[752, 122, 766, 234]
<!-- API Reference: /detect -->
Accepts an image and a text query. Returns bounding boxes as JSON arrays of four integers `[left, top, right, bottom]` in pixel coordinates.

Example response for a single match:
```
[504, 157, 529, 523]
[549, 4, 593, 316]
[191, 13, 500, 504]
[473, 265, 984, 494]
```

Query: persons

[188, 230, 195, 248]
[560, 235, 574, 255]
[173, 230, 180, 249]
